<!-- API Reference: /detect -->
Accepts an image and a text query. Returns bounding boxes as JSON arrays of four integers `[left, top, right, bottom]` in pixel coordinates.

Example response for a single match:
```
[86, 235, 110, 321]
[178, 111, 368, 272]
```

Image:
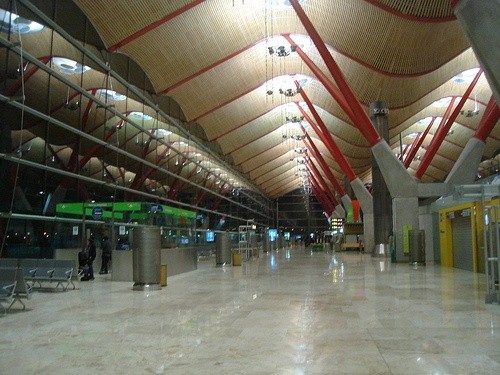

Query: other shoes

[99, 271, 103, 274]
[104, 272, 108, 274]
[89, 276, 95, 280]
[81, 277, 89, 280]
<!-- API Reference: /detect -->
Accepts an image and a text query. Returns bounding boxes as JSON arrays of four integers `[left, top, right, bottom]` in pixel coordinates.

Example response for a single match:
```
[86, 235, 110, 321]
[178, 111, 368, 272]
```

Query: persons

[78, 238, 97, 281]
[99, 236, 111, 274]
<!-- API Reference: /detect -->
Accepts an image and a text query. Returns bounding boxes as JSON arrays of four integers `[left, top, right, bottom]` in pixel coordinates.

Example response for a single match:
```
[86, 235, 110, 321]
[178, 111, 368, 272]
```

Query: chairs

[1, 258, 76, 312]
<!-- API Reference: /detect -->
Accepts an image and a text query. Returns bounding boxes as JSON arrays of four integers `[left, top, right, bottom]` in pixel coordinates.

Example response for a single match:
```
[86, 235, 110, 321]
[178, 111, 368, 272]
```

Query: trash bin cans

[232, 253, 241, 266]
[161, 264, 167, 286]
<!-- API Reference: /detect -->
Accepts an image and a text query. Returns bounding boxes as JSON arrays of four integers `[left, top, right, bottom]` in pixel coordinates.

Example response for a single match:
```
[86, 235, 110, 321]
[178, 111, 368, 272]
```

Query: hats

[88, 238, 94, 240]
